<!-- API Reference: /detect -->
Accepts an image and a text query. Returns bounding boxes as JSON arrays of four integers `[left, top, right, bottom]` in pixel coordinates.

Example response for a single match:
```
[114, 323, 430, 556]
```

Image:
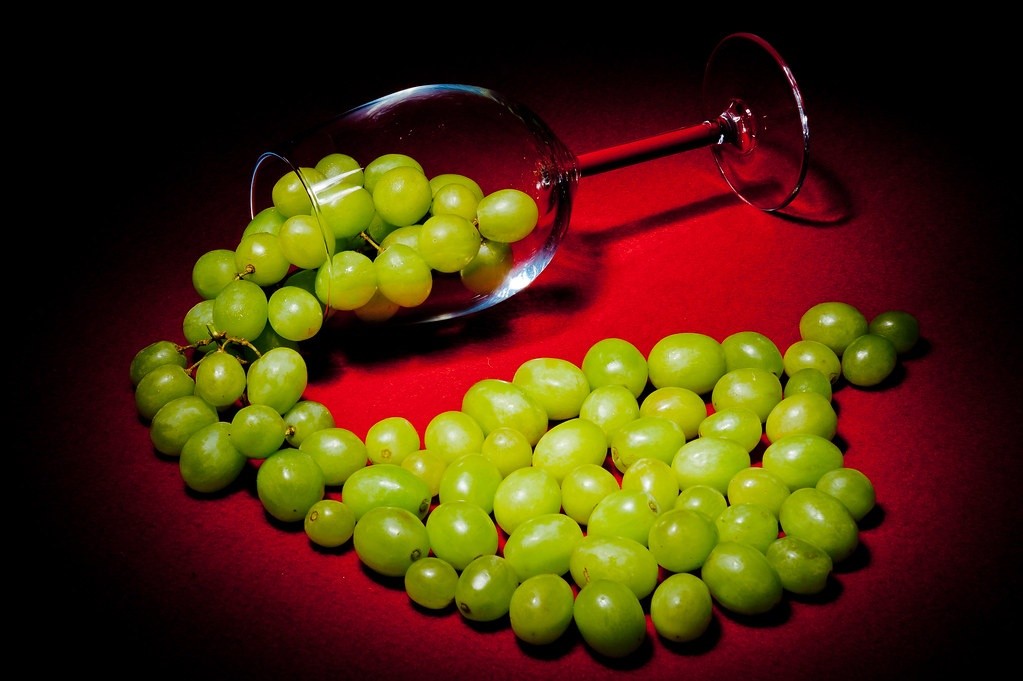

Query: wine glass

[249, 34, 810, 331]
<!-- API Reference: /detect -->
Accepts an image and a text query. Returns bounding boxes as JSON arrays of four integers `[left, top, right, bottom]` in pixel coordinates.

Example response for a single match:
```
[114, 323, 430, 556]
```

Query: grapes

[130, 302, 921, 654]
[183, 154, 537, 366]
